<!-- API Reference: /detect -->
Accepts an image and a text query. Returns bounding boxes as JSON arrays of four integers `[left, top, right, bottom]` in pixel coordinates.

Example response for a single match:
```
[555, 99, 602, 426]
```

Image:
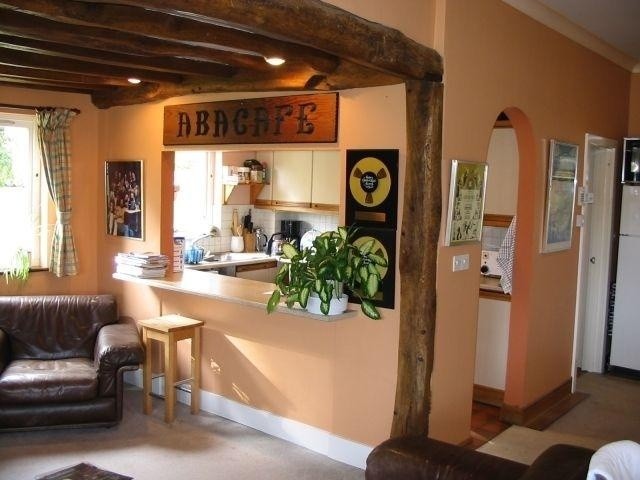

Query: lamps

[0, 293, 145, 433]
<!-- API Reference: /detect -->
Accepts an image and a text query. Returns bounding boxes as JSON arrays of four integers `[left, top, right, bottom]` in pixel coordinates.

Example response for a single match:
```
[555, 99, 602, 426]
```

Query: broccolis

[609, 186, 640, 370]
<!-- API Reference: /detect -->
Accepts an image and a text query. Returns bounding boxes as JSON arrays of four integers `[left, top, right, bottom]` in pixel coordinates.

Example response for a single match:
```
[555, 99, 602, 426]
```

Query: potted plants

[539, 138, 580, 253]
[443, 158, 490, 248]
[104, 160, 144, 241]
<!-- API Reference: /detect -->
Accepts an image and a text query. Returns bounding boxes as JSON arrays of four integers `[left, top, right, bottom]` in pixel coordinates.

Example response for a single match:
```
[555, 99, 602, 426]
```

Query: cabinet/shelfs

[253, 226, 267, 252]
[249, 164, 263, 184]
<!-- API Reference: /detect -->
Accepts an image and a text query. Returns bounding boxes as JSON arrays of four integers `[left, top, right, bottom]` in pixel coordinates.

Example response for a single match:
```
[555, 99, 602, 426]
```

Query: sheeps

[255, 228, 263, 251]
[191, 234, 216, 247]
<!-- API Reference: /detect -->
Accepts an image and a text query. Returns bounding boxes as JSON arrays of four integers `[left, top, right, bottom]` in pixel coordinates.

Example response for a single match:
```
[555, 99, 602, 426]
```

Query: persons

[108, 165, 142, 237]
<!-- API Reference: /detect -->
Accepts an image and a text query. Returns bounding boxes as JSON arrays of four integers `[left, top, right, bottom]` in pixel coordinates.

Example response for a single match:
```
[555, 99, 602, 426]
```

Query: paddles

[32, 464, 133, 480]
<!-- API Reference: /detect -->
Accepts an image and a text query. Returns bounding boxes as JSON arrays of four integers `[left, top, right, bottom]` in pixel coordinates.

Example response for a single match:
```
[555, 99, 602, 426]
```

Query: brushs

[280, 219, 300, 254]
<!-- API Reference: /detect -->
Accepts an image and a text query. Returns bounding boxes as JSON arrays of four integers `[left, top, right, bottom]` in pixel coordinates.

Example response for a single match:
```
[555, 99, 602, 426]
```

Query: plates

[474, 296, 511, 407]
[252, 152, 340, 216]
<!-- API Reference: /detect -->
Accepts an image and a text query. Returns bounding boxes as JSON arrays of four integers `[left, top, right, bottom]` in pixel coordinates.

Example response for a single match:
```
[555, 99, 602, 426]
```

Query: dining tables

[265, 221, 389, 320]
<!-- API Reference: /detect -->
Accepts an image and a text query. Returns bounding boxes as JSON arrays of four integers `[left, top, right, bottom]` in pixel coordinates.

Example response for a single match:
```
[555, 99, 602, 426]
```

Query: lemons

[265, 232, 297, 257]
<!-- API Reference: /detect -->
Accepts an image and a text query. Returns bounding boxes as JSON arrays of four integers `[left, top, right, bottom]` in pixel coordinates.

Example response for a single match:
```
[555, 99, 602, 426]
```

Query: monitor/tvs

[113, 247, 171, 279]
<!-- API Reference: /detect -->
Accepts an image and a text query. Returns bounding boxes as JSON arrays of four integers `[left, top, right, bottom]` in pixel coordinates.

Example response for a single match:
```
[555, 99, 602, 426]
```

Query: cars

[243, 232, 256, 253]
[231, 236, 244, 253]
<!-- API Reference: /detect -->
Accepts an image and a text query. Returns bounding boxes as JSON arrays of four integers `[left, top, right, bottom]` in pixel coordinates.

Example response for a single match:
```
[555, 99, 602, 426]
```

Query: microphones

[137, 315, 204, 424]
